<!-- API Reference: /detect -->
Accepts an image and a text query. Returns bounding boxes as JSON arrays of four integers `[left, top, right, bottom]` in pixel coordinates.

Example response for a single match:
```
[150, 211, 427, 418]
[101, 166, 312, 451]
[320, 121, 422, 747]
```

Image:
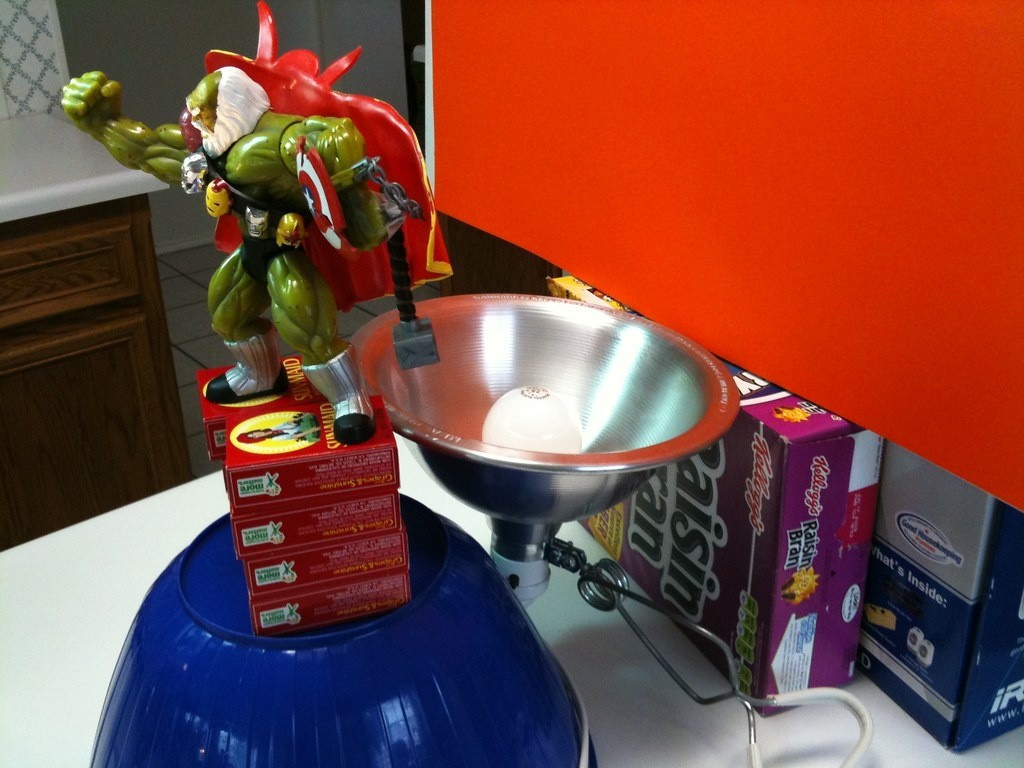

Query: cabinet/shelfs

[0, 199, 195, 554]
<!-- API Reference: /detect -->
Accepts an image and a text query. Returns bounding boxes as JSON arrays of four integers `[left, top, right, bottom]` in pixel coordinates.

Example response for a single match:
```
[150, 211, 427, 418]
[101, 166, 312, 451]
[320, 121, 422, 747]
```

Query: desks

[0, 431, 1024, 768]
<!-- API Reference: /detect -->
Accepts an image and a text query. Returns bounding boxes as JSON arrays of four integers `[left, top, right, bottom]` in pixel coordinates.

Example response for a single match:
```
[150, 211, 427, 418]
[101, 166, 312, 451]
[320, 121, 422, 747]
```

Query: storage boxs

[855, 434, 1023, 755]
[193, 366, 412, 640]
[542, 273, 885, 714]
[857, 441, 1023, 754]
